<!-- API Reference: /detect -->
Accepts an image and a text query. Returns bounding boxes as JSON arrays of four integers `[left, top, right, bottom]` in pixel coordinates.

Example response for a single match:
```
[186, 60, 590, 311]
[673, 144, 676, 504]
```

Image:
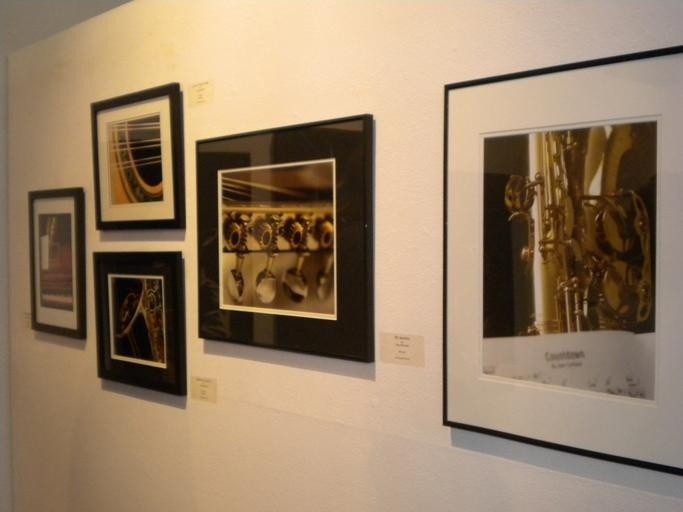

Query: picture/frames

[195, 114, 374, 363]
[92, 252, 186, 396]
[28, 187, 86, 342]
[90, 83, 185, 230]
[443, 45, 683, 477]
